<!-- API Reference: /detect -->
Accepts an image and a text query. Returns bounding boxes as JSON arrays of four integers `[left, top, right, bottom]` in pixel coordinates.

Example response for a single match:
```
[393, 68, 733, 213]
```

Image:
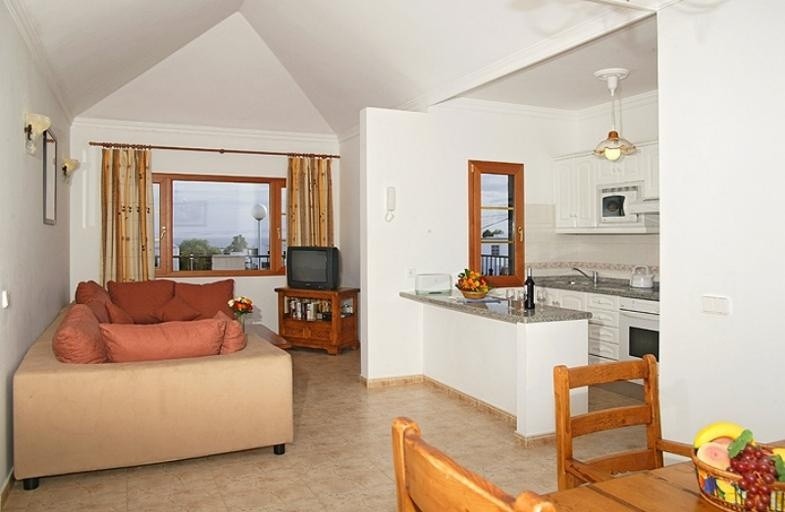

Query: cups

[505, 289, 526, 311]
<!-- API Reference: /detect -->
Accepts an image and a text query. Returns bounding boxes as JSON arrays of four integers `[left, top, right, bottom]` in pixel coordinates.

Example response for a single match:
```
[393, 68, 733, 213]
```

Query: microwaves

[599, 187, 640, 223]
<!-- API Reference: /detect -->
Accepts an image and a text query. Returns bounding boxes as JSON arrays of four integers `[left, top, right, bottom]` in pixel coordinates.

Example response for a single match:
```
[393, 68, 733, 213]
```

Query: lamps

[62, 158, 80, 183]
[592, 67, 636, 161]
[25, 113, 51, 155]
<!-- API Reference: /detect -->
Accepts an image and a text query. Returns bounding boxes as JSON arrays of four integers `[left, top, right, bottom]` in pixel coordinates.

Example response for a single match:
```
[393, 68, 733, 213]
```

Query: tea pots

[630, 265, 655, 288]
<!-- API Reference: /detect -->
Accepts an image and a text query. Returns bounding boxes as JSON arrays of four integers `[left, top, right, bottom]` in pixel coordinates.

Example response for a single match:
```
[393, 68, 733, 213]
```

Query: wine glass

[537, 289, 547, 312]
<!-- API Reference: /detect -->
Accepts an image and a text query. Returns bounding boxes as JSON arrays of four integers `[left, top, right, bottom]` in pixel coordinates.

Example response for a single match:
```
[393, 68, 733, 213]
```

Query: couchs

[12, 302, 295, 491]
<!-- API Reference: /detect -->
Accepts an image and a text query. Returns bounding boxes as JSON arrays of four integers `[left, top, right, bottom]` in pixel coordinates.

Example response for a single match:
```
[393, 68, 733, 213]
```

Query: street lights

[251, 202, 268, 254]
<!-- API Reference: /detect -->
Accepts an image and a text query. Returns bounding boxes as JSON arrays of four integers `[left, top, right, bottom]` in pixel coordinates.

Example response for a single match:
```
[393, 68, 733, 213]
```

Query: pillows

[51, 278, 247, 365]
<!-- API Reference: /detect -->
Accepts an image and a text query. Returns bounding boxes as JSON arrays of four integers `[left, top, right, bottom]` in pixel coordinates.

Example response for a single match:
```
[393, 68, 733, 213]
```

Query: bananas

[716, 448, 785, 504]
[693, 422, 756, 449]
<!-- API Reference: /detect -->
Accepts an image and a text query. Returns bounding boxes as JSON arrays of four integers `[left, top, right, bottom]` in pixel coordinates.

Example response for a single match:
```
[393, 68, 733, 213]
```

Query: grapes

[727, 446, 777, 512]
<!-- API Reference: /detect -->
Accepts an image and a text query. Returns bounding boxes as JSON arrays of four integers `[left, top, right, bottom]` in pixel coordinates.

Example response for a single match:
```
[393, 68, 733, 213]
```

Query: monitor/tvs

[288, 247, 338, 289]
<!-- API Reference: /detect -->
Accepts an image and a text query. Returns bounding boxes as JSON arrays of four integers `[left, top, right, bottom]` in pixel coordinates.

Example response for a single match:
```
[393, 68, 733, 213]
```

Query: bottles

[523, 266, 536, 309]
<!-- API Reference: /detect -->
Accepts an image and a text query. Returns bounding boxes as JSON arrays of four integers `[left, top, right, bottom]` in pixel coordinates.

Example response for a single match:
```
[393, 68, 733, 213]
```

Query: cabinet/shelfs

[587, 293, 618, 366]
[596, 142, 641, 185]
[532, 287, 561, 307]
[642, 144, 659, 201]
[561, 290, 588, 313]
[551, 152, 595, 234]
[275, 286, 361, 357]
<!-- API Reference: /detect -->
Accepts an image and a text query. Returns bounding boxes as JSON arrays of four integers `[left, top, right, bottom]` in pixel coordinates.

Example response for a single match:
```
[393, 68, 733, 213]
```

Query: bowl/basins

[455, 283, 494, 299]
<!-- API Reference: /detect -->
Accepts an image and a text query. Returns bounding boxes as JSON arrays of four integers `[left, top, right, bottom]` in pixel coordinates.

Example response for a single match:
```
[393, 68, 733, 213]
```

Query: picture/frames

[43, 127, 58, 226]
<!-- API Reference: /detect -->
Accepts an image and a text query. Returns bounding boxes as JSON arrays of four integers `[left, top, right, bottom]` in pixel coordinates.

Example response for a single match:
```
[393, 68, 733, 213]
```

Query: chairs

[553, 352, 699, 491]
[393, 417, 556, 512]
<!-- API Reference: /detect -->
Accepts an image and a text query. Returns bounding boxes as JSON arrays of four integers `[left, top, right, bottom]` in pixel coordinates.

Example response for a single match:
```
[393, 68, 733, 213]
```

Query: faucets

[572, 267, 598, 284]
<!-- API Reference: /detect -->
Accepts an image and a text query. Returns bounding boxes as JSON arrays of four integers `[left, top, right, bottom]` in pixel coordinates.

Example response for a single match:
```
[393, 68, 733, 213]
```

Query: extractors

[630, 198, 659, 216]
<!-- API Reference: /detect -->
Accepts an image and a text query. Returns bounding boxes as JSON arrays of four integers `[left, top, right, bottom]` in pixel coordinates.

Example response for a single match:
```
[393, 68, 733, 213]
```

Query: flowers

[226, 297, 253, 316]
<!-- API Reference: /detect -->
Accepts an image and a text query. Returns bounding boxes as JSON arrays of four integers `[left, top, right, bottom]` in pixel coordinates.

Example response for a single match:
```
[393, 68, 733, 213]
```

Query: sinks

[555, 278, 629, 288]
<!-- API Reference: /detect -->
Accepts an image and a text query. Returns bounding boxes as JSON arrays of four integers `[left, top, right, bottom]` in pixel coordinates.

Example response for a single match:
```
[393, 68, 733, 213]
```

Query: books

[286, 299, 353, 321]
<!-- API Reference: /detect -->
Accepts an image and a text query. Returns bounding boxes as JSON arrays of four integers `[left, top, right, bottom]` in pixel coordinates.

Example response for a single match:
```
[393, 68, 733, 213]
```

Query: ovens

[619, 310, 661, 389]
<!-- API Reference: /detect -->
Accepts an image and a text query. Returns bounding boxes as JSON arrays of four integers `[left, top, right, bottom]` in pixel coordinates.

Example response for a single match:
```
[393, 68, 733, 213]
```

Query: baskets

[691, 447, 784, 512]
